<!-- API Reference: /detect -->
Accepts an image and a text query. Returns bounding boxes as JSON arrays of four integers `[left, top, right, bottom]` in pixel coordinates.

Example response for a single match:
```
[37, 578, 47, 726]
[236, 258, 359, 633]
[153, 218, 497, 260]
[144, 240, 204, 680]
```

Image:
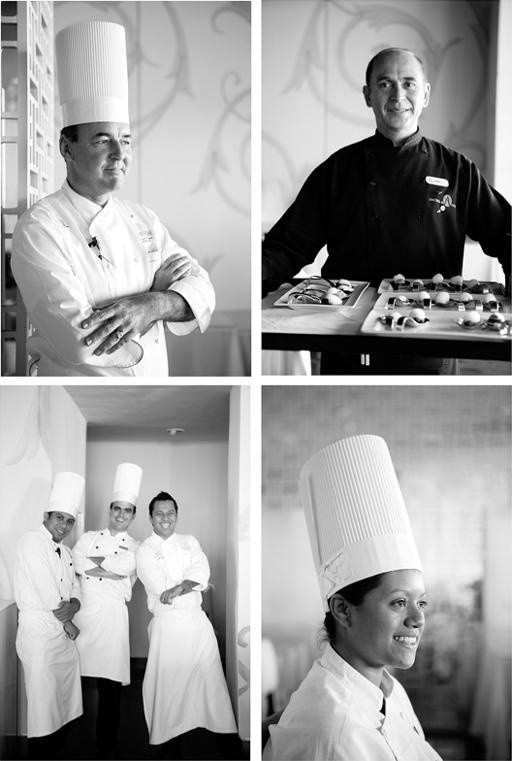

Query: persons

[262, 570, 443, 761]
[11, 122, 215, 376]
[262, 48, 512, 375]
[15, 491, 246, 761]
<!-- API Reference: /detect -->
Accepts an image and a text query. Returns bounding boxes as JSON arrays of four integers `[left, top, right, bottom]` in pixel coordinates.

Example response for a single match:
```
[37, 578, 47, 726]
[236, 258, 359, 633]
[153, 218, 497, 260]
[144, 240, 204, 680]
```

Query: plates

[271, 276, 512, 343]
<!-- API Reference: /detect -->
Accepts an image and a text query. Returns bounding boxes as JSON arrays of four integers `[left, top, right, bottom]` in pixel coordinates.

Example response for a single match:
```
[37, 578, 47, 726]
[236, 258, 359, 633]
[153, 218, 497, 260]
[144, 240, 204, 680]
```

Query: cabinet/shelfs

[2, 0, 54, 376]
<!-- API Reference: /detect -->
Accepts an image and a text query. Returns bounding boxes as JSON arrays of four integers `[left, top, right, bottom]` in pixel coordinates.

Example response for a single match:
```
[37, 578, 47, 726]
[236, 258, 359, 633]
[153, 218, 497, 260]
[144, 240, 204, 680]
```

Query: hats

[55, 21, 129, 132]
[299, 434, 424, 613]
[110, 462, 144, 508]
[45, 471, 81, 522]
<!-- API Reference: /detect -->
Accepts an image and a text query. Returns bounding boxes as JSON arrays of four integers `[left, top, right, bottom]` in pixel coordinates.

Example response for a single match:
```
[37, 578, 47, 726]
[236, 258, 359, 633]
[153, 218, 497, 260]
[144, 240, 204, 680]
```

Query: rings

[116, 330, 124, 339]
